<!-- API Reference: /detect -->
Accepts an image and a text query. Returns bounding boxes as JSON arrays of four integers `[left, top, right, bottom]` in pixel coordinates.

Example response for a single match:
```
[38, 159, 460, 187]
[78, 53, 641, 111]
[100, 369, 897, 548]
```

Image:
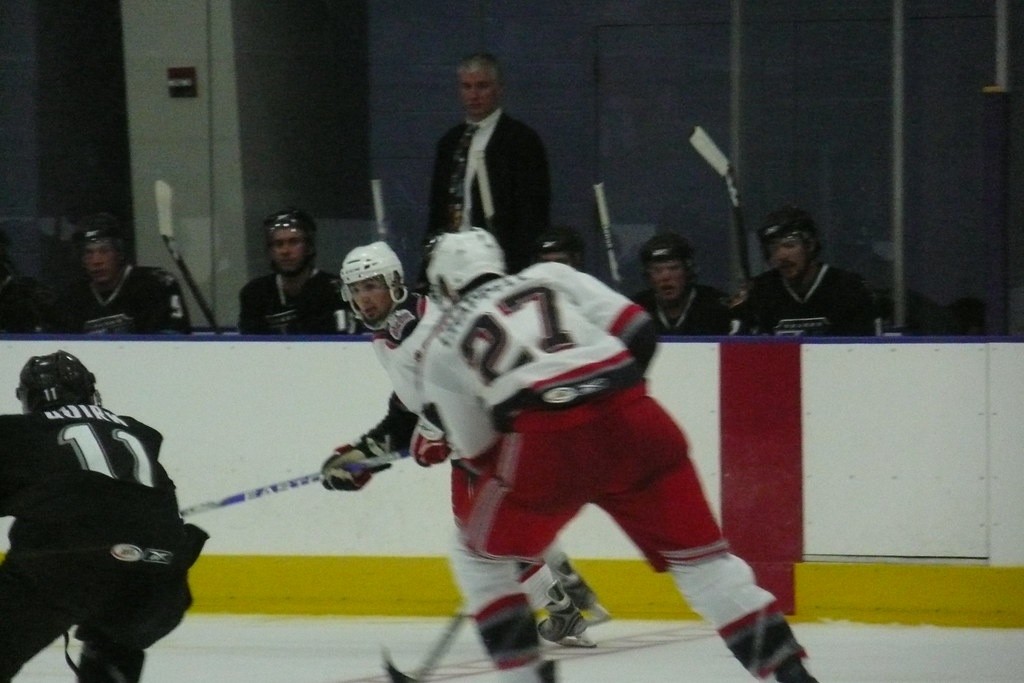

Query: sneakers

[536, 557, 608, 651]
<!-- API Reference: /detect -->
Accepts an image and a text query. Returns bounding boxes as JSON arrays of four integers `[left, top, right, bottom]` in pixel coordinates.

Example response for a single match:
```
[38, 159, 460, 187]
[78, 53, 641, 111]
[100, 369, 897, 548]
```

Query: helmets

[641, 234, 692, 262]
[425, 228, 505, 301]
[19, 350, 96, 411]
[757, 211, 817, 247]
[72, 211, 126, 254]
[533, 227, 583, 254]
[339, 242, 405, 312]
[265, 209, 313, 236]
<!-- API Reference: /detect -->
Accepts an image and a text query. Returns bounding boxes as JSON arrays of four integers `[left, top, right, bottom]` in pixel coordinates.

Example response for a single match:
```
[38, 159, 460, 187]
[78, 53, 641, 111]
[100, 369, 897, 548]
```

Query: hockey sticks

[179, 447, 412, 518]
[369, 176, 388, 242]
[687, 123, 757, 334]
[382, 612, 464, 683]
[153, 179, 221, 335]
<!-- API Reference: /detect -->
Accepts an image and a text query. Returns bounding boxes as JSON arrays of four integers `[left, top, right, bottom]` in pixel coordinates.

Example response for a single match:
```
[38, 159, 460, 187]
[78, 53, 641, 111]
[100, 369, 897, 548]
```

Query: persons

[0, 211, 192, 334]
[417, 52, 551, 283]
[238, 209, 374, 336]
[411, 225, 817, 683]
[0, 350, 210, 683]
[323, 241, 598, 640]
[540, 207, 878, 337]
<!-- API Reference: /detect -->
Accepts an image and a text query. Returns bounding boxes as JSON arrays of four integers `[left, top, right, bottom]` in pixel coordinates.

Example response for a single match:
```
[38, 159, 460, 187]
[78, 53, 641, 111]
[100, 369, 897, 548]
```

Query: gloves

[409, 414, 448, 466]
[322, 444, 373, 492]
